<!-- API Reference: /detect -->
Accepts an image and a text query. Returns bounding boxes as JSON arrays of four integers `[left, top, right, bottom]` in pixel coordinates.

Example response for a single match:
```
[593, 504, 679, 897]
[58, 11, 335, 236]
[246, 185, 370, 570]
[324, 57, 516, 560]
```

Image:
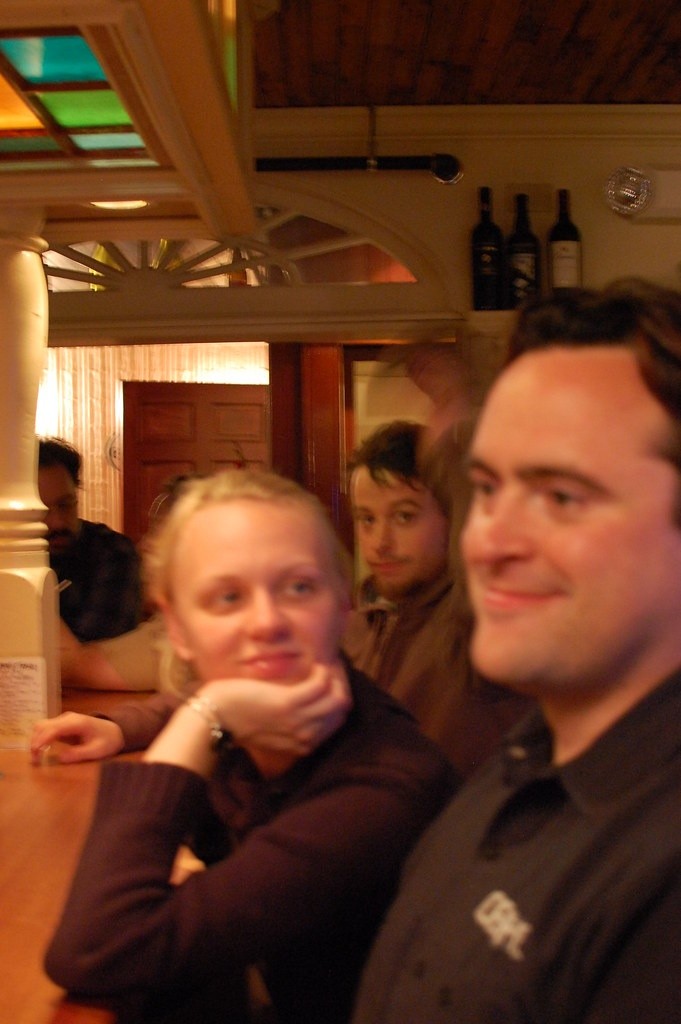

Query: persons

[30, 419, 497, 767]
[42, 469, 462, 1024]
[352, 276, 681, 1024]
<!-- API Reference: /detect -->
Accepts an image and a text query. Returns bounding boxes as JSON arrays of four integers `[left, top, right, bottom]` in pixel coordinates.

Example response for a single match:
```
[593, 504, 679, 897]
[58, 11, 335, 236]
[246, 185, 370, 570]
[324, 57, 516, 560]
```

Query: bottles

[507, 193, 540, 301]
[471, 187, 503, 311]
[546, 190, 581, 299]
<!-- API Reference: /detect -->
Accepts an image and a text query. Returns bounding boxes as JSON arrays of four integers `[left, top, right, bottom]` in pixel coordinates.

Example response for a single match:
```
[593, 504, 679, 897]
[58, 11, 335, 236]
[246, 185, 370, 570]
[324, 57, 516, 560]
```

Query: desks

[0, 687, 204, 1023]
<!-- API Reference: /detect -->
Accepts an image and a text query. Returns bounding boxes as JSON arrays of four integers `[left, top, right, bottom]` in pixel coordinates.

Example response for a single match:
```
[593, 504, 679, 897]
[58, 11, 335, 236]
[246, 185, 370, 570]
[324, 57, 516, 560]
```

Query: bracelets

[185, 691, 223, 742]
